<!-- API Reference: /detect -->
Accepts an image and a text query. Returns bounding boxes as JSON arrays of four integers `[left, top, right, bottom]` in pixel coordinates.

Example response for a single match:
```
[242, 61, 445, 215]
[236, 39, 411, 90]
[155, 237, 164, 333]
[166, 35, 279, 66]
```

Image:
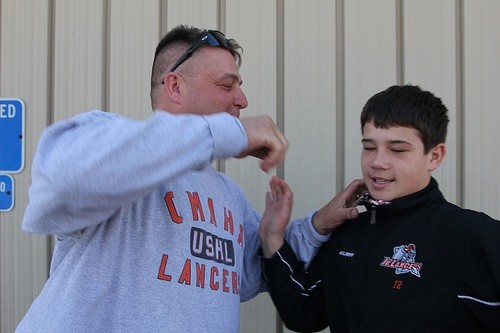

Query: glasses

[162, 30, 227, 84]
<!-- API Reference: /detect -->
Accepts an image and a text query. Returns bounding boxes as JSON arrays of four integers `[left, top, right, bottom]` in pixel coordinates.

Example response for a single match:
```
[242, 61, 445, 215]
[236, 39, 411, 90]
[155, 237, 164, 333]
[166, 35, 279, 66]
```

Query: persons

[12, 23, 369, 333]
[259, 82, 500, 333]
